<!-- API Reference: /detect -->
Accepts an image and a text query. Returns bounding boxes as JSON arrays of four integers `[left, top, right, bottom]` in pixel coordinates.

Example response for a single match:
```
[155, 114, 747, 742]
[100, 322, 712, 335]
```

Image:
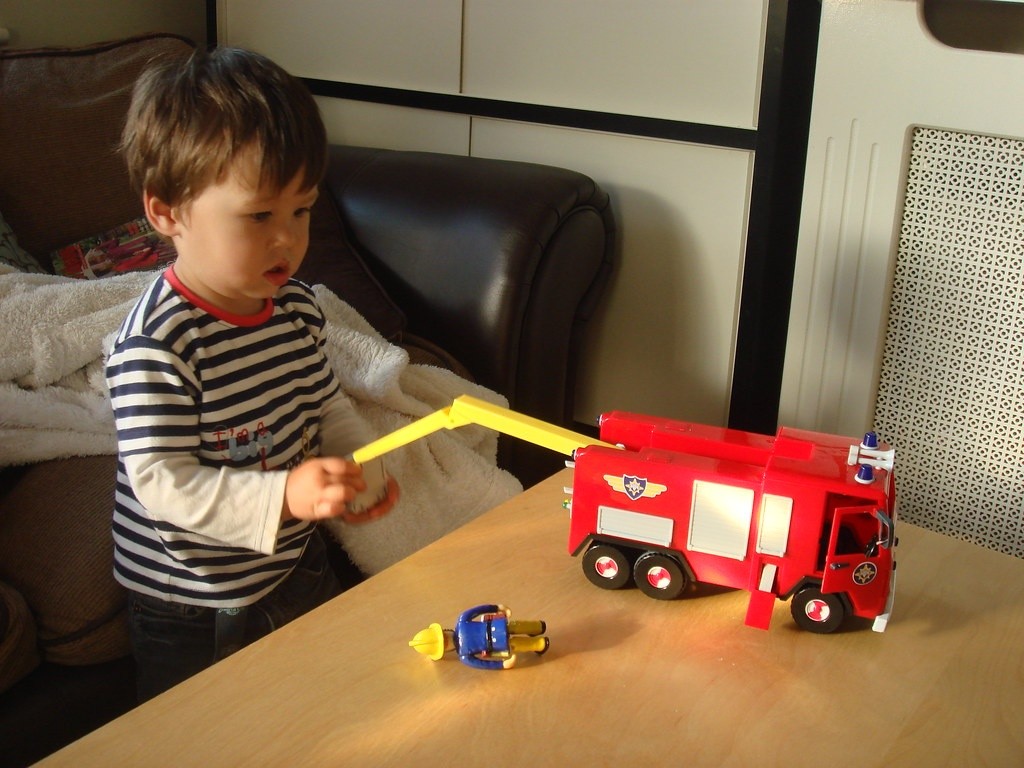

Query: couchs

[0, 29, 615, 768]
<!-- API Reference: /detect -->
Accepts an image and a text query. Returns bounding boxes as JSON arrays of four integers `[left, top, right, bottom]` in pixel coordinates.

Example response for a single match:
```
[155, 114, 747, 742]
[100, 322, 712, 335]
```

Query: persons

[107, 47, 389, 706]
[408, 604, 550, 669]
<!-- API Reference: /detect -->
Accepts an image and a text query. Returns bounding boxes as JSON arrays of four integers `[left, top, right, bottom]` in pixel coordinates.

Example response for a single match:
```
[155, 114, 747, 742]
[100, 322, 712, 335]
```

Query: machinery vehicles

[349, 394, 901, 636]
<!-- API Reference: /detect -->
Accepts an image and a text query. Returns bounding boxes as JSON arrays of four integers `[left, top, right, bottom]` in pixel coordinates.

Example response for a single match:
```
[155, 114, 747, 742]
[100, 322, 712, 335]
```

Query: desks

[29, 466, 1024, 768]
